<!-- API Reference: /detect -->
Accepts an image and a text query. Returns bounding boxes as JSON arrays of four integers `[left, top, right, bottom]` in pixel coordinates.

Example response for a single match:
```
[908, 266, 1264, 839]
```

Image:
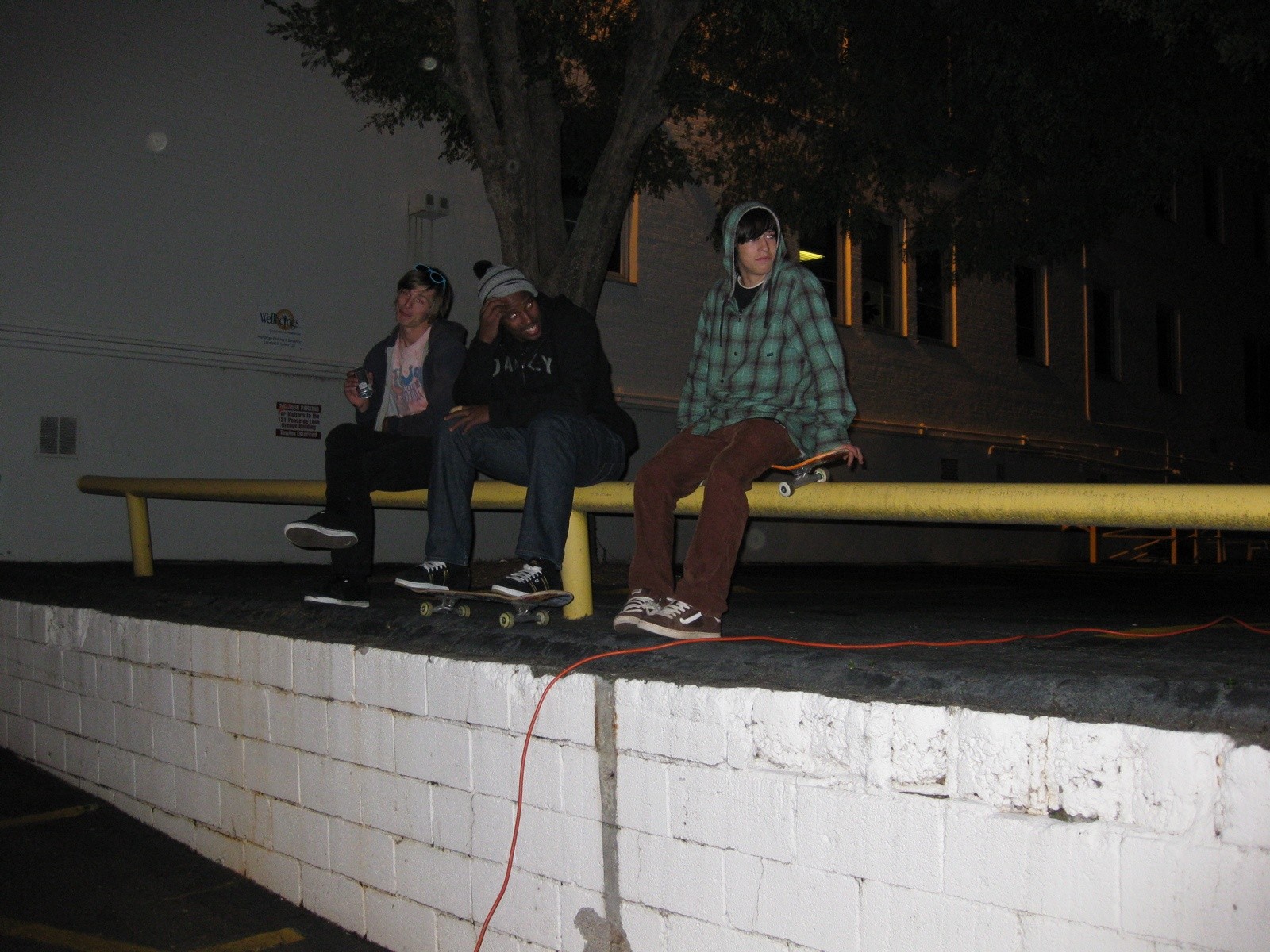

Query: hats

[474, 260, 539, 305]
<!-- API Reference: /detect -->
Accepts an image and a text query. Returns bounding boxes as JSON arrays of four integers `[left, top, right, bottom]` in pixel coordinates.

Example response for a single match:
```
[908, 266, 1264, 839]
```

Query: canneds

[352, 366, 374, 399]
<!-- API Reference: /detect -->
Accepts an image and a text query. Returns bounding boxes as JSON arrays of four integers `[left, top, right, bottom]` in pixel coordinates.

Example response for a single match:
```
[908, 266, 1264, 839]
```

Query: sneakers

[637, 598, 721, 640]
[284, 509, 358, 549]
[491, 559, 561, 597]
[394, 559, 472, 592]
[613, 590, 658, 634]
[303, 577, 370, 608]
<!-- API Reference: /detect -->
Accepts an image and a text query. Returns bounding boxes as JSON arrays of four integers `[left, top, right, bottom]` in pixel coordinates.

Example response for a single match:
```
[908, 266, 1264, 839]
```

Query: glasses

[415, 263, 446, 293]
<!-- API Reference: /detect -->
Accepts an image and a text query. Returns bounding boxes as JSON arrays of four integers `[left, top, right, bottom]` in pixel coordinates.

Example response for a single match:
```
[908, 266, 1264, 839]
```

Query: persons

[395, 260, 640, 598]
[613, 201, 863, 641]
[285, 263, 470, 608]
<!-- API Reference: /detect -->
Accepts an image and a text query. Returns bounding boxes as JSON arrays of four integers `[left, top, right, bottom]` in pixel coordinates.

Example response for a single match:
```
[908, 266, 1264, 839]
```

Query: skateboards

[771, 447, 853, 498]
[407, 586, 575, 628]
